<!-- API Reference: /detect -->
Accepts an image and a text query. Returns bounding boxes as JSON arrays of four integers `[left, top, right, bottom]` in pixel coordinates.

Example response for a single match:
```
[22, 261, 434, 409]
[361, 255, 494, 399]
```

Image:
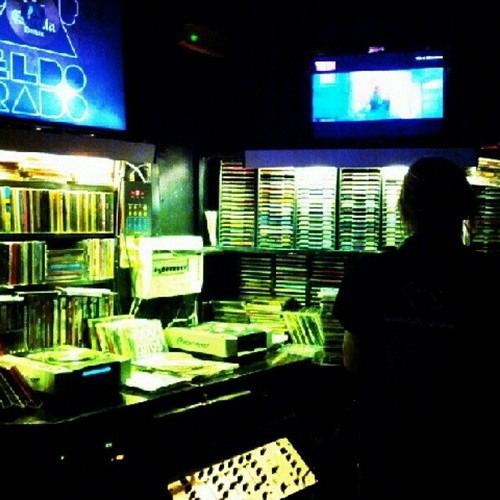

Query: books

[0, 156, 500, 411]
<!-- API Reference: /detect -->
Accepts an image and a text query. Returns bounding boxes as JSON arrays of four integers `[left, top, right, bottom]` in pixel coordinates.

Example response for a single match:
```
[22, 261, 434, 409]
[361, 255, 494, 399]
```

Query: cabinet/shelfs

[0, 128, 156, 317]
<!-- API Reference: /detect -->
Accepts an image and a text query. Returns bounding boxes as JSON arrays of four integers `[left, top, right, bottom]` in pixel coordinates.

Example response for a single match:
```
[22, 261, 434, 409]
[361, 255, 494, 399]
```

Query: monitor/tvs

[0, 0, 128, 132]
[313, 50, 446, 136]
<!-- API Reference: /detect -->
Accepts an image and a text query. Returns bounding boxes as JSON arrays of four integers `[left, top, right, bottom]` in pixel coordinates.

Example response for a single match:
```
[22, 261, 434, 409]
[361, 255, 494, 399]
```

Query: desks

[0, 342, 327, 500]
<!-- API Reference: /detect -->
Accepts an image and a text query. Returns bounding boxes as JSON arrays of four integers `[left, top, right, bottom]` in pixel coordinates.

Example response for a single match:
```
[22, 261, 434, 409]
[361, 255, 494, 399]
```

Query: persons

[331, 157, 500, 373]
[356, 83, 391, 114]
